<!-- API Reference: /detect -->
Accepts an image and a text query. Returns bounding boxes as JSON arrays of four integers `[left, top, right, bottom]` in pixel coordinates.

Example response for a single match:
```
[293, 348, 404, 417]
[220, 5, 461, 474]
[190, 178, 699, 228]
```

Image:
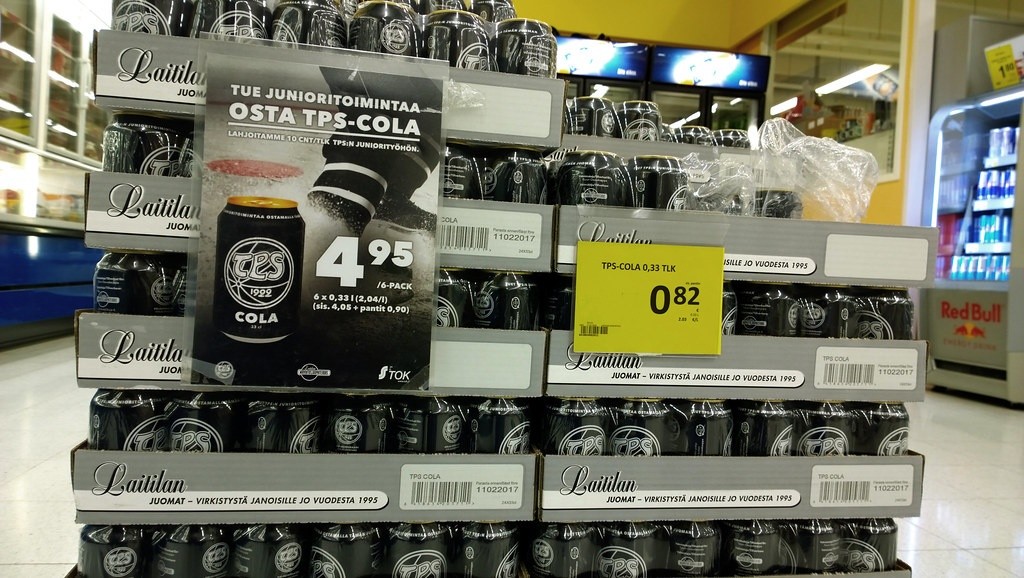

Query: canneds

[77, 0, 919, 578]
[950, 126, 1020, 281]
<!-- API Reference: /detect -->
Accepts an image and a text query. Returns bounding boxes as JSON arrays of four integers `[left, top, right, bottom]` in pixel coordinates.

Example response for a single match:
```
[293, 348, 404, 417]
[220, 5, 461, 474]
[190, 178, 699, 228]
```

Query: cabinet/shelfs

[0, 0, 114, 350]
[63, 30, 939, 578]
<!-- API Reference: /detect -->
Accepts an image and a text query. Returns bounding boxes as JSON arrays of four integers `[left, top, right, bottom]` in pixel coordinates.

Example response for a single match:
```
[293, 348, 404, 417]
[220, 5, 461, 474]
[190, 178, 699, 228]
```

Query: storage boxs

[805, 104, 866, 143]
